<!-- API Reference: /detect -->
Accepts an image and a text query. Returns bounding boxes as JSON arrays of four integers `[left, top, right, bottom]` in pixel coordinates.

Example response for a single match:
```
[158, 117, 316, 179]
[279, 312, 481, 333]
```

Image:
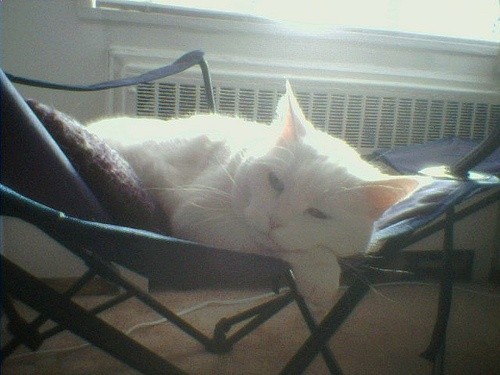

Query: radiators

[109, 44, 499, 298]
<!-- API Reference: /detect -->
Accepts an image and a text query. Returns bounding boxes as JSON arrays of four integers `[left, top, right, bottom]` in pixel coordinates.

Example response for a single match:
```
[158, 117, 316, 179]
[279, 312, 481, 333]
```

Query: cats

[84, 80, 433, 299]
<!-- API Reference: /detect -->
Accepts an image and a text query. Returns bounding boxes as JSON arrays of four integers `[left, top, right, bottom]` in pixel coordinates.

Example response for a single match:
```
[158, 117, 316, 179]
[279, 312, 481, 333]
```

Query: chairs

[0, 71, 500, 374]
